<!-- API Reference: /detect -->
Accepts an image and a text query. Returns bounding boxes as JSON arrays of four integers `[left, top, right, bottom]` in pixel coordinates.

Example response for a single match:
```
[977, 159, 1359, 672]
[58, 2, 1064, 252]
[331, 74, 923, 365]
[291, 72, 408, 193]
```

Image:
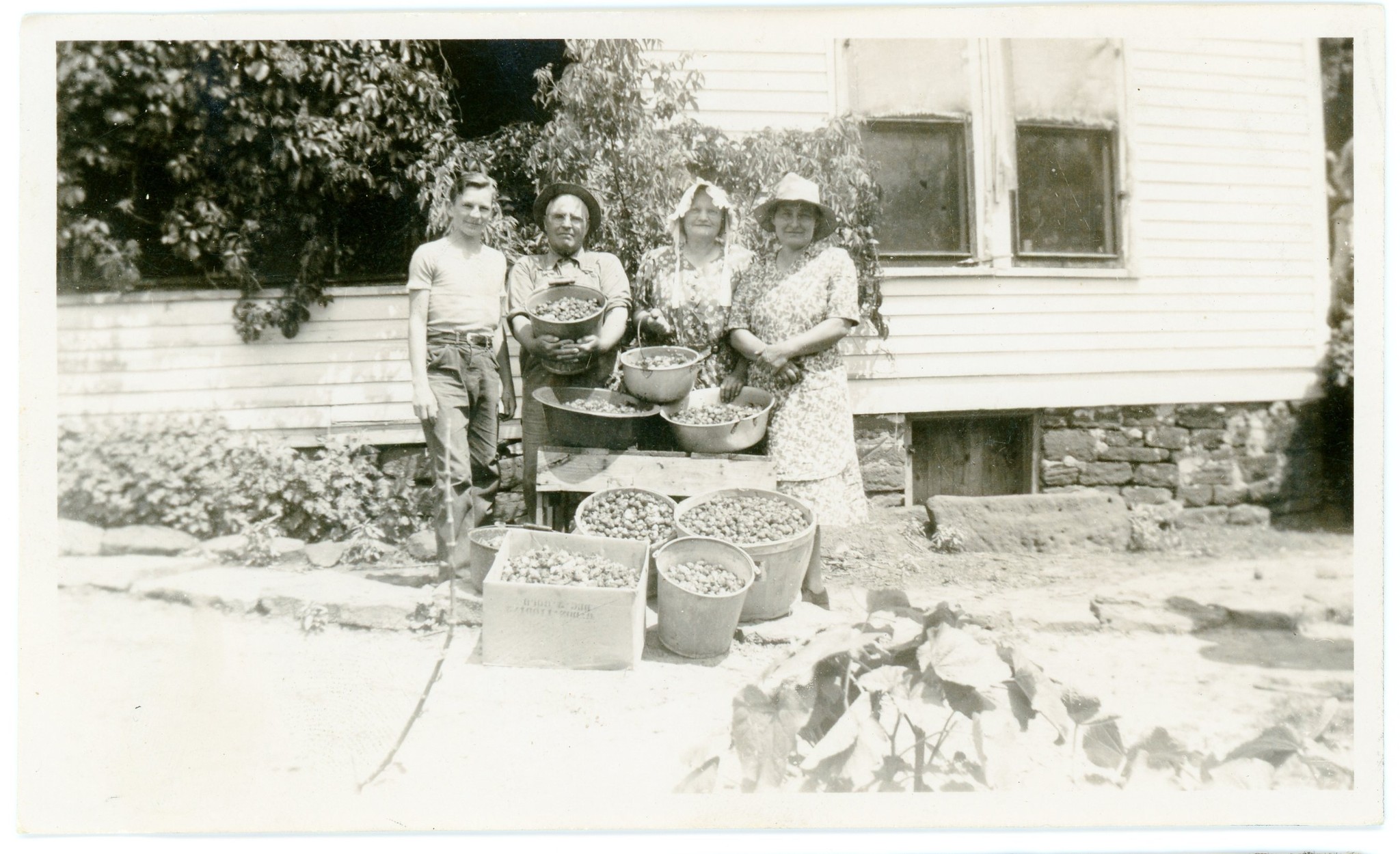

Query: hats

[664, 177, 739, 308]
[753, 176, 837, 242]
[533, 183, 601, 237]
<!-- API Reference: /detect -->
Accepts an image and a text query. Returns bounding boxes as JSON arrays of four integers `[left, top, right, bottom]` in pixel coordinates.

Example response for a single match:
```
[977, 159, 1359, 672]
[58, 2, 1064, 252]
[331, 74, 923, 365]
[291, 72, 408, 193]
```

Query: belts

[427, 332, 493, 349]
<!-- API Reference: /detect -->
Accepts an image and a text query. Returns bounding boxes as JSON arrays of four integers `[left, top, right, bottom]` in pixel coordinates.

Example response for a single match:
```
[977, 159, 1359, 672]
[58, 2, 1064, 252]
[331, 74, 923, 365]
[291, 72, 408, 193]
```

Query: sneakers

[800, 586, 830, 611]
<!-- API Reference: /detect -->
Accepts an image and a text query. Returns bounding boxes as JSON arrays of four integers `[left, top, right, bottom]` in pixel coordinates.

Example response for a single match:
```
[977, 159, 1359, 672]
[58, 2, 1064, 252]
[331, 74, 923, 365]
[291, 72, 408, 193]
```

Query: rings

[720, 383, 725, 390]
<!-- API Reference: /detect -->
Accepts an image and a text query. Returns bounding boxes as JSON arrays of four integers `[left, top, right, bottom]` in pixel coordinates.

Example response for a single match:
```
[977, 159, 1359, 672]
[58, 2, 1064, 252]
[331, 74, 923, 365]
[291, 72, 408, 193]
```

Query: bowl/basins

[531, 385, 660, 447]
[657, 385, 775, 454]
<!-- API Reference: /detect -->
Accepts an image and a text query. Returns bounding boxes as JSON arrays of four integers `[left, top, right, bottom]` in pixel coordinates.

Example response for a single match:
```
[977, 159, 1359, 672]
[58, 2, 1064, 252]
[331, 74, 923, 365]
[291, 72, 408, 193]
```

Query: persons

[406, 171, 515, 585]
[632, 179, 759, 452]
[506, 182, 635, 532]
[726, 172, 868, 601]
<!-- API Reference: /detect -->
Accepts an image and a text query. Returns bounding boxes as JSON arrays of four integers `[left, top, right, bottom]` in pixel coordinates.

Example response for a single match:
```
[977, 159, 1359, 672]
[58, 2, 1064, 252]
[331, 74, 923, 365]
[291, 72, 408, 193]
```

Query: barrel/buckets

[576, 483, 818, 660]
[528, 283, 606, 375]
[469, 523, 530, 594]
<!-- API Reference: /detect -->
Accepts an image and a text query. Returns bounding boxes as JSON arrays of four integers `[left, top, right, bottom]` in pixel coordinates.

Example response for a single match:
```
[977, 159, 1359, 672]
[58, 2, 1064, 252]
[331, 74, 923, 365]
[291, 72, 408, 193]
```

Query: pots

[622, 347, 701, 405]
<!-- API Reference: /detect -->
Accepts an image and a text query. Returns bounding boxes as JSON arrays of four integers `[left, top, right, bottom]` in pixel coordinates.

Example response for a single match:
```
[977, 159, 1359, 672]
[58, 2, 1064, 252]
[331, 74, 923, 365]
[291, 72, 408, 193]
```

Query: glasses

[687, 206, 722, 217]
[544, 213, 590, 227]
